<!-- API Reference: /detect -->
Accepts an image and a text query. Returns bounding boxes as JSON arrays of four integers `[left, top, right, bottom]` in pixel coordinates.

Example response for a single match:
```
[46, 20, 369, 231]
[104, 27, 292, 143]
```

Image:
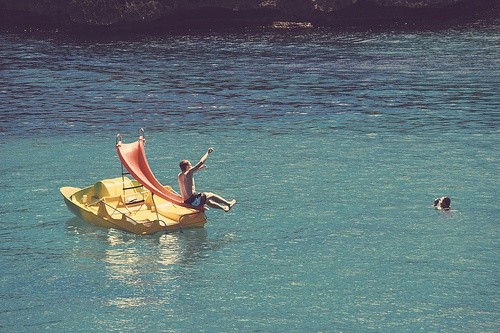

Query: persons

[434, 196, 452, 210]
[176, 147, 236, 212]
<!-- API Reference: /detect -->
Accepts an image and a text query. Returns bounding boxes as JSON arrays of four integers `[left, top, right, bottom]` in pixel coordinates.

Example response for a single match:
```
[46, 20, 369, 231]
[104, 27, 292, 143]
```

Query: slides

[115, 136, 210, 212]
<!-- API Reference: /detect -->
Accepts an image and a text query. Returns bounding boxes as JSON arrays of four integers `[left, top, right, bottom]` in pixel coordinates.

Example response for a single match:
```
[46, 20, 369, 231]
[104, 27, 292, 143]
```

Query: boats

[59, 128, 208, 232]
